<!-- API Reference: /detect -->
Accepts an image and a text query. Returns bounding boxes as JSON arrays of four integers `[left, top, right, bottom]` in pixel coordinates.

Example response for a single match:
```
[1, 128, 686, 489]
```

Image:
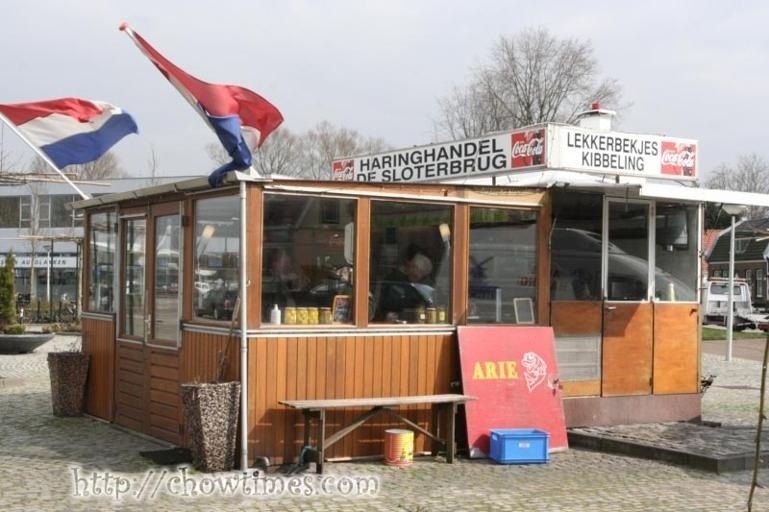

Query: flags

[1, 95, 139, 172]
[121, 23, 285, 191]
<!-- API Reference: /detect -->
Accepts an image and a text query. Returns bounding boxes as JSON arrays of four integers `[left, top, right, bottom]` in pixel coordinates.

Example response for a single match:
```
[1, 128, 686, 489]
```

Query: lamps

[437, 223, 451, 249]
[197, 226, 214, 256]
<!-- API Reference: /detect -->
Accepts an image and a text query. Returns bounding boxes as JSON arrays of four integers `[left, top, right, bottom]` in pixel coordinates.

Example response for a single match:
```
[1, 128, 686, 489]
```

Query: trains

[470, 227, 694, 299]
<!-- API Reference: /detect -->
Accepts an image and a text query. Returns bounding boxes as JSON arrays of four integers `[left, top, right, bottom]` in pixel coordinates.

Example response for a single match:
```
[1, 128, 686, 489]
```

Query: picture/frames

[321, 198, 340, 224]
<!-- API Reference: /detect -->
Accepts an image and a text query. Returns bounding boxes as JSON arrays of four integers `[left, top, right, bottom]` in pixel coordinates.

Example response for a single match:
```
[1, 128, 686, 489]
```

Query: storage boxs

[489, 428, 550, 463]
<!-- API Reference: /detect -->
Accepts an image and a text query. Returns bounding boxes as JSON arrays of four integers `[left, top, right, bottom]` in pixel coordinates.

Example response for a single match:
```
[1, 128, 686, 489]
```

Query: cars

[93, 263, 144, 308]
[197, 289, 235, 319]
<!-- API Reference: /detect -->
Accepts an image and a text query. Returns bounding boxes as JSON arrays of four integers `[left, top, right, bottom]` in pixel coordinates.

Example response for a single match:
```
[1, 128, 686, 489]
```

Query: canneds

[437, 309, 446, 323]
[296, 307, 309, 325]
[283, 307, 296, 324]
[318, 307, 332, 324]
[426, 308, 437, 324]
[306, 308, 319, 325]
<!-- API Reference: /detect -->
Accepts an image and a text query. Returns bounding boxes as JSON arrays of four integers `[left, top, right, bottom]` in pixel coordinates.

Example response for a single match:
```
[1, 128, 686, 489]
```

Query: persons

[374, 253, 435, 320]
[261, 248, 298, 292]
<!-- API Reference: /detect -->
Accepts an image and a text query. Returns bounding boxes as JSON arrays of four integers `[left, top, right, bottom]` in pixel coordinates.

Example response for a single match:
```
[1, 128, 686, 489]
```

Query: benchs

[280, 393, 480, 474]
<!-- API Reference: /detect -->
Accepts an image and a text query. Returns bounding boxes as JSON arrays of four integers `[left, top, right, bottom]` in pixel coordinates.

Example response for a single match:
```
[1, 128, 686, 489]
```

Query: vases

[47, 353, 90, 416]
[179, 380, 242, 472]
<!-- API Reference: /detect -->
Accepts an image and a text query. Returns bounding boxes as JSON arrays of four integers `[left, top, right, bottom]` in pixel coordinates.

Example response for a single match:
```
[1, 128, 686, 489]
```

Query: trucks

[706, 278, 769, 330]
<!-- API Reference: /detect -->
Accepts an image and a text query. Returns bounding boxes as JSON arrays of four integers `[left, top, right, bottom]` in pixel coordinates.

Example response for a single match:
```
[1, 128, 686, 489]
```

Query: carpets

[139, 448, 191, 466]
